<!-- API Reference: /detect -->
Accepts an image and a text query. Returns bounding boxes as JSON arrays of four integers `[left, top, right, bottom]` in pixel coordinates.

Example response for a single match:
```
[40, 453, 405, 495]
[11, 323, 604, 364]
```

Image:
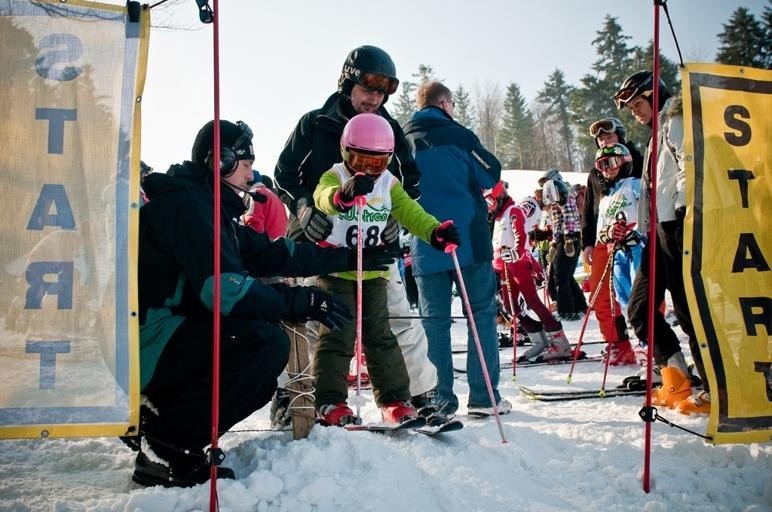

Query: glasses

[595, 156, 632, 171]
[590, 121, 624, 137]
[343, 65, 398, 95]
[613, 73, 654, 109]
[341, 144, 393, 175]
[439, 100, 455, 108]
[483, 181, 503, 208]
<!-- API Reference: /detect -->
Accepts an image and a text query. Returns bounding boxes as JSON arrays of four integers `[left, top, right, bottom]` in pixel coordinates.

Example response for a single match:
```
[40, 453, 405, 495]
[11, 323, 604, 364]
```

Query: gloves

[431, 221, 460, 253]
[348, 243, 403, 271]
[334, 172, 374, 212]
[296, 210, 333, 243]
[565, 236, 575, 257]
[546, 241, 558, 261]
[608, 222, 636, 241]
[304, 286, 353, 330]
[675, 206, 686, 253]
[501, 248, 519, 263]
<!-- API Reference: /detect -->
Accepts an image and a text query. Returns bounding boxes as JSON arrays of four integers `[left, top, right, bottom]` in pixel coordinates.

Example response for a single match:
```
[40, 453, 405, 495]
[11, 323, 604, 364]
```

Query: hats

[192, 120, 254, 165]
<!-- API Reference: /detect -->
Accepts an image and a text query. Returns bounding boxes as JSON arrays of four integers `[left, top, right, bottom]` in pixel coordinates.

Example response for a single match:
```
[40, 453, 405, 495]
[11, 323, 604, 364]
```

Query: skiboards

[313, 417, 467, 438]
[518, 383, 665, 402]
[453, 356, 608, 372]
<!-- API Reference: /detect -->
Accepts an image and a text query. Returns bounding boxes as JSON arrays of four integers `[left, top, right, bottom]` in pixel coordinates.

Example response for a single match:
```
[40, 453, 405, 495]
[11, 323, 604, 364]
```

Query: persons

[346, 228, 419, 386]
[240, 170, 289, 241]
[400, 82, 512, 421]
[580, 117, 665, 366]
[595, 142, 662, 384]
[482, 168, 589, 363]
[312, 112, 462, 426]
[120, 121, 404, 489]
[613, 70, 710, 415]
[270, 45, 449, 427]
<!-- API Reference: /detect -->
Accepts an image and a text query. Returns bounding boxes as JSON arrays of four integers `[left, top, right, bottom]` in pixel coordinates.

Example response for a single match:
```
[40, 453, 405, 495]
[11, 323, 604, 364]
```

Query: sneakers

[269, 389, 293, 429]
[315, 390, 512, 426]
[120, 405, 234, 487]
[602, 341, 713, 416]
[498, 312, 589, 363]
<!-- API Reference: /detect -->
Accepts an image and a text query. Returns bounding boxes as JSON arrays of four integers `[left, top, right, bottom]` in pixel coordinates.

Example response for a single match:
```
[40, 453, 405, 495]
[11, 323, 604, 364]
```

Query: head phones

[204, 120, 254, 177]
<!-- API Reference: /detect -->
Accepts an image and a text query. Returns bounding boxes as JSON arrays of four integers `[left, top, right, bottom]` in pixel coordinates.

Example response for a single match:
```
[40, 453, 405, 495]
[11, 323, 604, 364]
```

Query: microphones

[221, 177, 268, 204]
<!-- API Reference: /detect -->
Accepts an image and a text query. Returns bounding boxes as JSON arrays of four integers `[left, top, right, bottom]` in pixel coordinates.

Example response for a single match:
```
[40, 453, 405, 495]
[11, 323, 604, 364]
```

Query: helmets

[596, 143, 634, 182]
[482, 180, 508, 211]
[543, 179, 567, 205]
[342, 114, 396, 160]
[594, 118, 625, 148]
[339, 46, 396, 103]
[620, 71, 667, 128]
